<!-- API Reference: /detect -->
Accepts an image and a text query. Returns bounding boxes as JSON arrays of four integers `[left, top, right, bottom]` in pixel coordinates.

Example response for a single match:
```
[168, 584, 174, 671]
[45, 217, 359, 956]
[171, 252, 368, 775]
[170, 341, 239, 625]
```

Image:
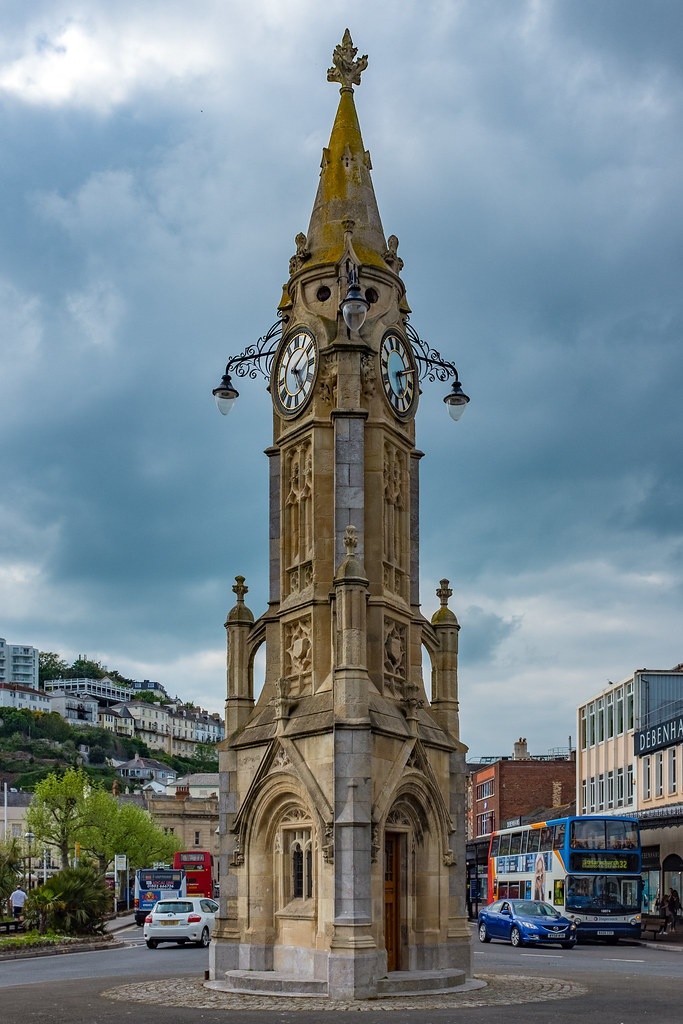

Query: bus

[135, 868, 197, 929]
[464, 831, 526, 904]
[173, 850, 214, 901]
[486, 816, 650, 946]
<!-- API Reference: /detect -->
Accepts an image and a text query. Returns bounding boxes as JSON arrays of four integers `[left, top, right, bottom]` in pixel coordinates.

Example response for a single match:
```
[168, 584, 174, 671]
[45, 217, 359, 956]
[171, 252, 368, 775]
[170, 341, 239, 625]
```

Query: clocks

[379, 325, 419, 424]
[271, 323, 319, 420]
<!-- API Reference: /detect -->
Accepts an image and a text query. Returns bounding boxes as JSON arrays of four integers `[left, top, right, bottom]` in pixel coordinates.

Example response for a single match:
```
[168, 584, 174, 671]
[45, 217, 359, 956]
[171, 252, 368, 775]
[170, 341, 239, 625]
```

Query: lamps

[336, 262, 371, 333]
[412, 339, 471, 421]
[212, 328, 282, 415]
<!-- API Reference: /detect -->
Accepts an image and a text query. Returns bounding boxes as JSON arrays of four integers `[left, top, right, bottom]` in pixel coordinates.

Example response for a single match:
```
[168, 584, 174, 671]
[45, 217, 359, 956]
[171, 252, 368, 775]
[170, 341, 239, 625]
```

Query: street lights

[25, 833, 47, 887]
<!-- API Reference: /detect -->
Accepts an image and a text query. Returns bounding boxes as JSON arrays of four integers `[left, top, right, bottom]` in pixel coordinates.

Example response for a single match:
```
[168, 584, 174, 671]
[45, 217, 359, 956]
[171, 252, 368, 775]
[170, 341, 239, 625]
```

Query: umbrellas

[15, 870, 37, 881]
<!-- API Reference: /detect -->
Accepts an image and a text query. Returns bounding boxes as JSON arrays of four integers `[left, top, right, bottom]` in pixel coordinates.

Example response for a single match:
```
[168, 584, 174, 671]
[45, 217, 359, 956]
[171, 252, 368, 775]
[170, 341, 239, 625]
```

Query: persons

[570, 839, 585, 849]
[502, 887, 518, 899]
[603, 882, 621, 903]
[612, 838, 636, 850]
[529, 903, 547, 915]
[493, 833, 565, 856]
[568, 883, 581, 896]
[535, 853, 544, 901]
[507, 904, 510, 910]
[658, 887, 683, 935]
[9, 880, 28, 933]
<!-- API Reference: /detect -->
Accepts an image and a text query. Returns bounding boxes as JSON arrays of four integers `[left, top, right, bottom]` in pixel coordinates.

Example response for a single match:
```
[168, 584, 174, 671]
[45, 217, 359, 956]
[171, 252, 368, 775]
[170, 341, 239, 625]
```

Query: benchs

[641, 915, 670, 941]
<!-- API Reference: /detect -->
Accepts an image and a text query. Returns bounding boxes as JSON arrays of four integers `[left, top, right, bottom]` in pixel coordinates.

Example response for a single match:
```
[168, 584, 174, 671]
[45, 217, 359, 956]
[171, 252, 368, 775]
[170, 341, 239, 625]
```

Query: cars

[478, 898, 578, 949]
[142, 897, 220, 950]
